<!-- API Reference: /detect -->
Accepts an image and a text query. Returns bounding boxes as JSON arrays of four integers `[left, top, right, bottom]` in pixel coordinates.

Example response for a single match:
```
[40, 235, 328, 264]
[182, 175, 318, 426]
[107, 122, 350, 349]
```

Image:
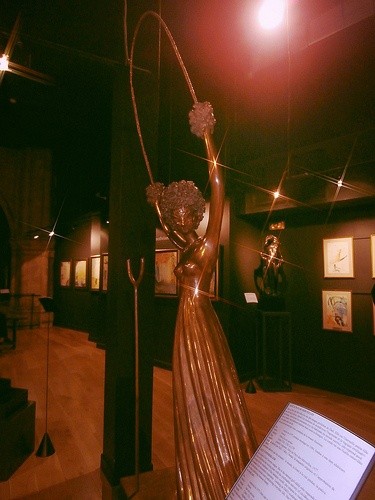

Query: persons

[147, 100, 259, 500]
[259, 234, 283, 296]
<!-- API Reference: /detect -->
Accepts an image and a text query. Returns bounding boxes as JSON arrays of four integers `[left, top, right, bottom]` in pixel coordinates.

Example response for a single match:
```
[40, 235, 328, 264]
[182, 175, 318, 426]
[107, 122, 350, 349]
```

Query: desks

[254, 307, 293, 392]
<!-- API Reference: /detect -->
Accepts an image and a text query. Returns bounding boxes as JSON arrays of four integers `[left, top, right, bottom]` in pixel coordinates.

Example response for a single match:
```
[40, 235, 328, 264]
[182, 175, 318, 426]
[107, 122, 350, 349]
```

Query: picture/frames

[209, 259, 218, 302]
[58, 252, 108, 294]
[322, 291, 353, 333]
[323, 239, 354, 278]
[155, 248, 181, 298]
